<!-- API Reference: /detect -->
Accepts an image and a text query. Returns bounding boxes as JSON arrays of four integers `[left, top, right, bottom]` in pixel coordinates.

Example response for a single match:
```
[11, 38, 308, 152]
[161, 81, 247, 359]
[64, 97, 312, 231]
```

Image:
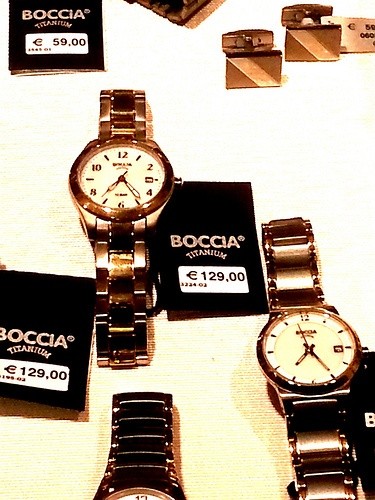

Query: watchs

[69, 88, 183, 369]
[96, 391, 185, 500]
[257, 214, 369, 500]
